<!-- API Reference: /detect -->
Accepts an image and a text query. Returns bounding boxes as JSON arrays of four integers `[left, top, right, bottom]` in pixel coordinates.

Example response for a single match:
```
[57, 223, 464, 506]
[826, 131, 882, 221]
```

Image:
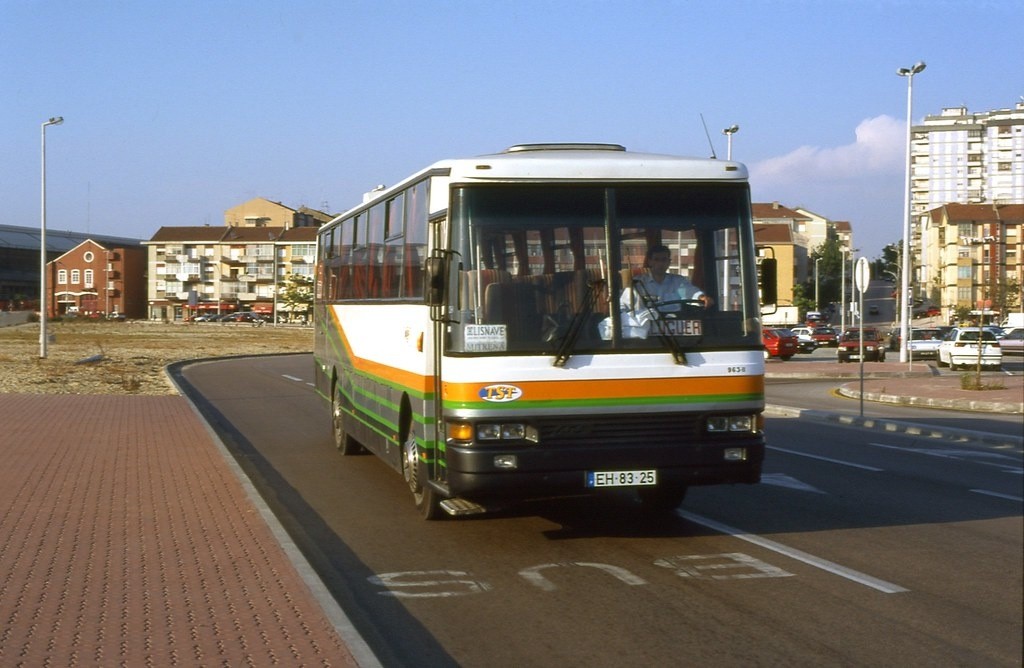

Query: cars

[910, 310, 927, 319]
[988, 328, 1007, 339]
[837, 327, 885, 363]
[182, 312, 287, 323]
[89, 313, 103, 318]
[936, 327, 1002, 371]
[914, 298, 923, 307]
[812, 328, 837, 347]
[790, 328, 814, 348]
[927, 309, 940, 317]
[887, 327, 918, 350]
[908, 329, 944, 360]
[869, 306, 879, 314]
[761, 327, 796, 361]
[997, 328, 1024, 350]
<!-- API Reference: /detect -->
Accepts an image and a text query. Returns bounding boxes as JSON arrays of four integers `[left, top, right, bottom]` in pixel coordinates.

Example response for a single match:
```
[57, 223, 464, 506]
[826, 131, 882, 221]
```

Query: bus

[311, 141, 768, 522]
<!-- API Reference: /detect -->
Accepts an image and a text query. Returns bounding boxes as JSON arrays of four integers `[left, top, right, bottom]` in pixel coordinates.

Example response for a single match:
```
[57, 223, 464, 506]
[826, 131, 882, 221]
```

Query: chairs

[461, 267, 650, 342]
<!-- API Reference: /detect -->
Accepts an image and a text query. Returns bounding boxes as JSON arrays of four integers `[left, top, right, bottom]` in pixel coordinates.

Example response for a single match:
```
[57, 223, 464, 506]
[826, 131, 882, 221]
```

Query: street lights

[39, 117, 65, 357]
[894, 61, 928, 362]
[851, 248, 859, 329]
[721, 124, 741, 160]
[815, 256, 824, 313]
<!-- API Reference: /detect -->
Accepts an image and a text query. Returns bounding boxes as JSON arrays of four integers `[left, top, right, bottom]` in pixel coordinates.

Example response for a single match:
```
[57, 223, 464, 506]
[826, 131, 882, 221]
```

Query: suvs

[110, 311, 125, 321]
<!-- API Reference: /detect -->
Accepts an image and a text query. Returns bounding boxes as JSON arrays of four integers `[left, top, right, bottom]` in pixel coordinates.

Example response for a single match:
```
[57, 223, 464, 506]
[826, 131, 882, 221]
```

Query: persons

[620, 246, 712, 338]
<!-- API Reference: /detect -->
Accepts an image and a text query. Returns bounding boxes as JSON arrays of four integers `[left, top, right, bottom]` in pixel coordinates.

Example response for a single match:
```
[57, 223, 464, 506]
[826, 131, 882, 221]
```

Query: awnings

[255, 304, 273, 313]
[277, 303, 309, 312]
